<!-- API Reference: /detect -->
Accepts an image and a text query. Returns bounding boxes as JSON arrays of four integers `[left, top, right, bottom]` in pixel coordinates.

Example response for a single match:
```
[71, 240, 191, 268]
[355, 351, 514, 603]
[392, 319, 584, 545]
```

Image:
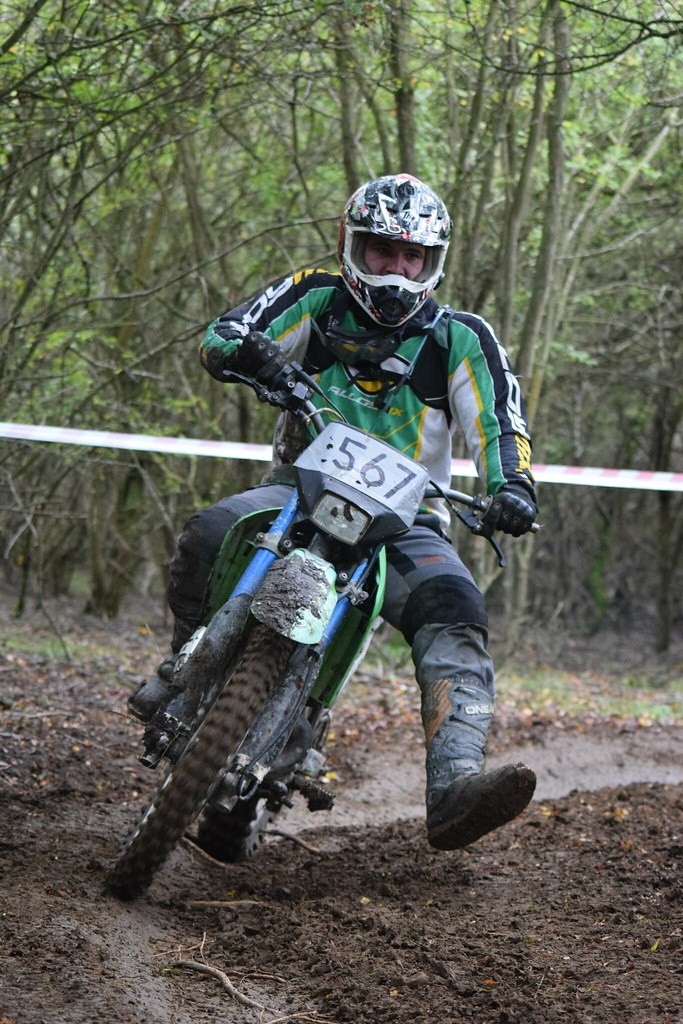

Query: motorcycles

[99, 323, 540, 903]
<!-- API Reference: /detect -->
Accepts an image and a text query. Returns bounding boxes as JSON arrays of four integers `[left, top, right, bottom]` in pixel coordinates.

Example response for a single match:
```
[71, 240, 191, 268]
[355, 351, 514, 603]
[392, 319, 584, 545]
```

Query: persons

[123, 172, 538, 850]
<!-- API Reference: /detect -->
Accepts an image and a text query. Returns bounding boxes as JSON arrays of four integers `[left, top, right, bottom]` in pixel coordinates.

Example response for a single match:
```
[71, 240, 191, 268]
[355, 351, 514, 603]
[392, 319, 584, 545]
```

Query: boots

[411, 622, 536, 850]
[133, 582, 203, 715]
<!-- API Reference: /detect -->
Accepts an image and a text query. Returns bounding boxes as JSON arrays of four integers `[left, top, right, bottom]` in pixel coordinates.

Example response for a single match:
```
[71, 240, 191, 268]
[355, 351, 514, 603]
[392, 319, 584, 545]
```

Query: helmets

[338, 173, 453, 327]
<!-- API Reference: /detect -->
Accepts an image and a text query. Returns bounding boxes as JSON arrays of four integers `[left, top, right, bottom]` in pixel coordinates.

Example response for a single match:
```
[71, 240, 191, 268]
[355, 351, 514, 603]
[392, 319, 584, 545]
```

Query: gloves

[225, 331, 289, 403]
[480, 484, 539, 537]
